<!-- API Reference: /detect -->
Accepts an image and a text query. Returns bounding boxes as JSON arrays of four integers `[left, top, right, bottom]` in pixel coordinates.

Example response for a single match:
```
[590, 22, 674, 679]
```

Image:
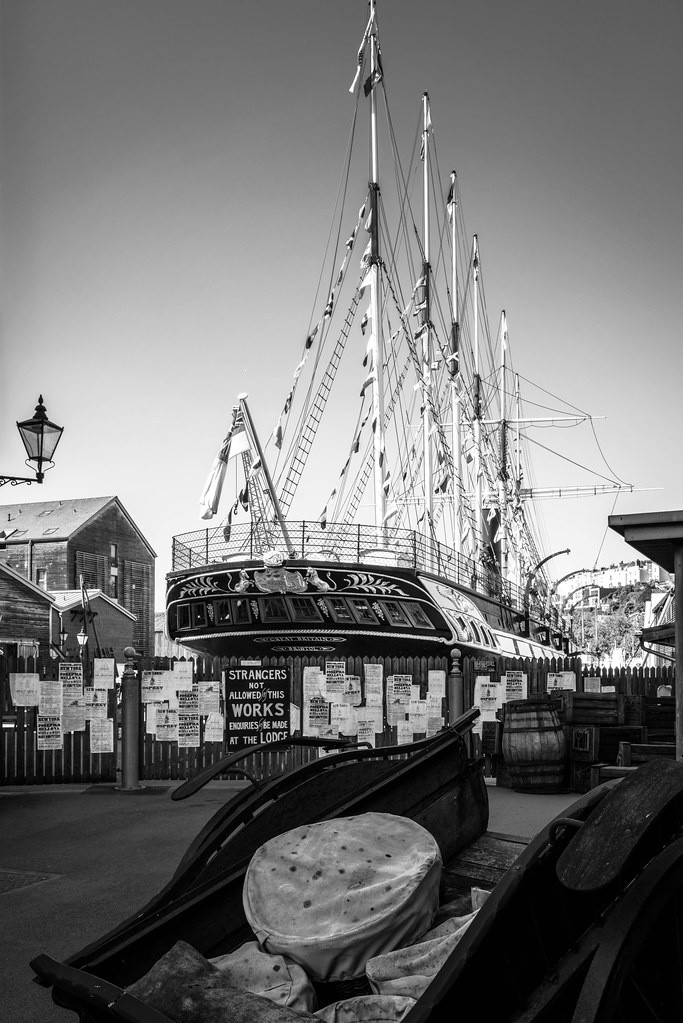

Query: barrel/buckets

[502, 700, 566, 793]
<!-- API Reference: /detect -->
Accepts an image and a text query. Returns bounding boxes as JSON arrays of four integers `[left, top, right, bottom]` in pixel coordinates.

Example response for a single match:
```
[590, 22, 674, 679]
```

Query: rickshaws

[31, 703, 683, 1023]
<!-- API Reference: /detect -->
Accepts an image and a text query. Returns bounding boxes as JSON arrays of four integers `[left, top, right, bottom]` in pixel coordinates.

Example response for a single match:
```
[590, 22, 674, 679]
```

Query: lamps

[51, 626, 68, 648]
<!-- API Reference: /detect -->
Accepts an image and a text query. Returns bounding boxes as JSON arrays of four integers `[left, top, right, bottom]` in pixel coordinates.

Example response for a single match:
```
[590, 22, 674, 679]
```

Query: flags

[200, 6, 545, 581]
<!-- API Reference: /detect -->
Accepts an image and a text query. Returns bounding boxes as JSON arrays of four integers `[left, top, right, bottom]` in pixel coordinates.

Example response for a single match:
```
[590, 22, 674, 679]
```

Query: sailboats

[165, 0, 663, 662]
